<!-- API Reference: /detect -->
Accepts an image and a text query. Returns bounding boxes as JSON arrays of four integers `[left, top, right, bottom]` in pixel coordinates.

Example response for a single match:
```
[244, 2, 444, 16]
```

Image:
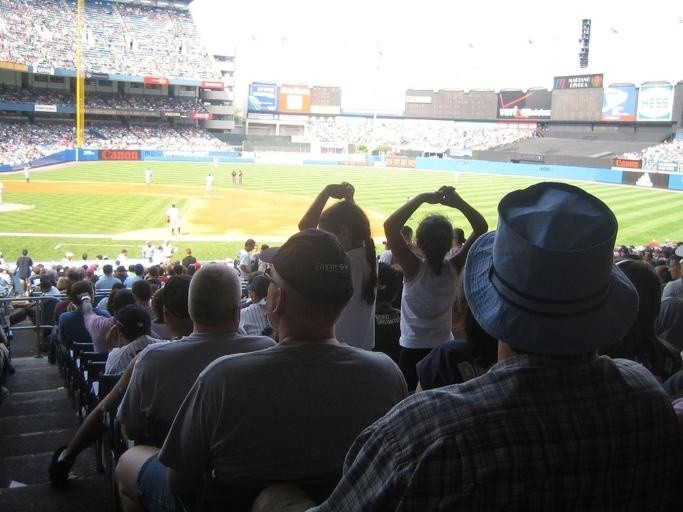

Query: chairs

[59, 341, 128, 474]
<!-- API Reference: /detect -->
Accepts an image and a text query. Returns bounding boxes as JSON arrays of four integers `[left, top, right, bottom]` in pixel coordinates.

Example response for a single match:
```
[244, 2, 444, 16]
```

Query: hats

[464, 182, 639, 356]
[247, 228, 353, 302]
[115, 305, 151, 336]
[161, 275, 191, 316]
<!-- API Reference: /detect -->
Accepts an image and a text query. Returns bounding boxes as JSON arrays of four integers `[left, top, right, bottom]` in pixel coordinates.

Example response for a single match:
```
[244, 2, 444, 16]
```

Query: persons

[1, 1, 243, 190]
[303, 115, 534, 168]
[1, 182, 683, 512]
[620, 139, 682, 173]
